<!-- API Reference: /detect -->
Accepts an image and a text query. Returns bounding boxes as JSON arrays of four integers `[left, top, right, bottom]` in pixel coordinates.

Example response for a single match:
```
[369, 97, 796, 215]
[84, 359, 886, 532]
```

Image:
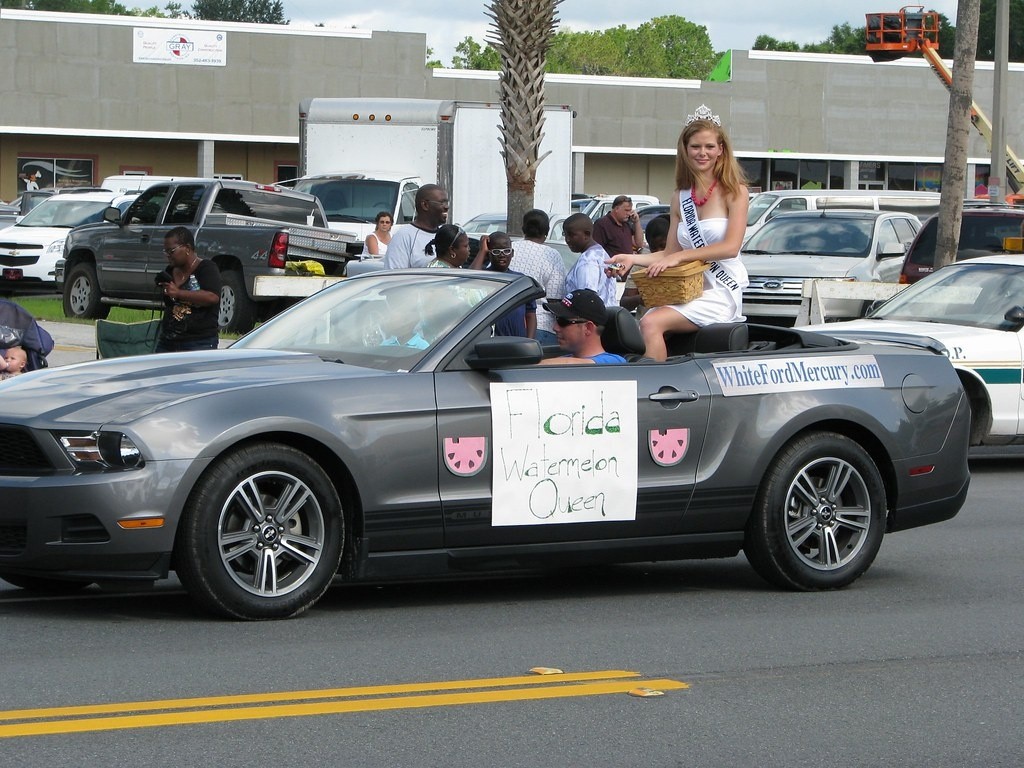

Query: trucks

[292, 94, 573, 255]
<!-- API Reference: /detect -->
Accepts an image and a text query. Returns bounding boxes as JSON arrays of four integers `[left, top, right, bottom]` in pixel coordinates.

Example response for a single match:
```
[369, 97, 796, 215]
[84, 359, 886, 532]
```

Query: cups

[306, 215, 315, 226]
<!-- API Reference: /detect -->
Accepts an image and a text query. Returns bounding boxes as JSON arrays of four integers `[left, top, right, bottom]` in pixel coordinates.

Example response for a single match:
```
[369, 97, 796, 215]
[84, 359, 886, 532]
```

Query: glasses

[553, 313, 600, 329]
[614, 197, 632, 209]
[422, 200, 449, 207]
[488, 247, 514, 256]
[161, 242, 188, 256]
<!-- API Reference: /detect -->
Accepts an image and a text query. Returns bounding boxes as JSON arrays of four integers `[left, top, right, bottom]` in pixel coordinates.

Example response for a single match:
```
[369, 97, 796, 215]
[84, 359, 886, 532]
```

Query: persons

[468, 231, 539, 340]
[620, 214, 679, 321]
[361, 211, 394, 261]
[384, 184, 451, 270]
[602, 104, 750, 363]
[155, 227, 224, 354]
[378, 302, 431, 350]
[593, 196, 646, 281]
[0, 347, 27, 386]
[562, 214, 618, 307]
[533, 289, 627, 366]
[486, 208, 567, 344]
[422, 224, 485, 339]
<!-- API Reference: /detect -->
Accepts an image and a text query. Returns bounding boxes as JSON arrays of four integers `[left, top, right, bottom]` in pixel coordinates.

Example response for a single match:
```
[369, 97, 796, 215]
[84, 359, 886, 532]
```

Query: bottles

[189, 275, 202, 307]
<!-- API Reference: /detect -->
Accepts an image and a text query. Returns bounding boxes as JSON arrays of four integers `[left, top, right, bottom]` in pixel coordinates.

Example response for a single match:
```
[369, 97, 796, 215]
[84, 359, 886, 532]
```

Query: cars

[738, 208, 924, 329]
[571, 192, 670, 235]
[789, 254, 1024, 447]
[456, 212, 570, 245]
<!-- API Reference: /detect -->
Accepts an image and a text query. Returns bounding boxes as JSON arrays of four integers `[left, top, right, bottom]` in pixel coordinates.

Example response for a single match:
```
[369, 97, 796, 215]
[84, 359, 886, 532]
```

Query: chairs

[599, 306, 654, 363]
[95, 317, 163, 359]
[322, 190, 347, 209]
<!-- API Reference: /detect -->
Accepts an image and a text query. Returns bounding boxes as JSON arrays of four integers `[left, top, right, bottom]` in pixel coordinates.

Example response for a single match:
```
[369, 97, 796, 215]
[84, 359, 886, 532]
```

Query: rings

[164, 288, 167, 292]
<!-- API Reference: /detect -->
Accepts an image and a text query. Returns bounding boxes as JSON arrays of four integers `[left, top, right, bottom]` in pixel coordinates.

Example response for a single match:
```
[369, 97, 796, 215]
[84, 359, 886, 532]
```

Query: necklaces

[691, 179, 718, 206]
[177, 256, 199, 280]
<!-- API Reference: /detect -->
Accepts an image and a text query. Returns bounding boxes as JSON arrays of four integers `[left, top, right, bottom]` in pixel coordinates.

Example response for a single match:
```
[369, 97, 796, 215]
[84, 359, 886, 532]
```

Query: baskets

[629, 259, 711, 308]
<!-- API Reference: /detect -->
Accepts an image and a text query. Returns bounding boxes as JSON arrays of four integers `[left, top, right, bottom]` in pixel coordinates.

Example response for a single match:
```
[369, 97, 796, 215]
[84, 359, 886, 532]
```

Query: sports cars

[0, 267, 972, 619]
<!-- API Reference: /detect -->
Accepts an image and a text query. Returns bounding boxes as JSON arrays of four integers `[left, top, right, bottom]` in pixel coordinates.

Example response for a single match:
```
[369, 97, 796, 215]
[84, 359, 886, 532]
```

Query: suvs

[898, 201, 1024, 287]
[0, 190, 148, 296]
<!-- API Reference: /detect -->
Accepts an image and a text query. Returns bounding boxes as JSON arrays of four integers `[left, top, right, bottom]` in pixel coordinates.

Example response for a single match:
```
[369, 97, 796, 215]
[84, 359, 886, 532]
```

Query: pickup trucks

[0, 187, 113, 234]
[63, 179, 363, 337]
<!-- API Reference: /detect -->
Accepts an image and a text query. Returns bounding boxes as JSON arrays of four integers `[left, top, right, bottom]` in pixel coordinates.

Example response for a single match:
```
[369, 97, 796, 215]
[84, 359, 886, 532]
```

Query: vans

[101, 175, 211, 196]
[739, 188, 941, 247]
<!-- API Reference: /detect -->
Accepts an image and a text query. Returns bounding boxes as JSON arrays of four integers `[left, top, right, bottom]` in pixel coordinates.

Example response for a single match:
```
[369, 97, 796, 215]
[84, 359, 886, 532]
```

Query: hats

[543, 289, 609, 329]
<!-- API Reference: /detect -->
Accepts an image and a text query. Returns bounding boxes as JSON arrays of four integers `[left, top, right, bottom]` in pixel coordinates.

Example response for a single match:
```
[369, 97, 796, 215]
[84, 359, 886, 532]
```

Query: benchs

[665, 324, 749, 351]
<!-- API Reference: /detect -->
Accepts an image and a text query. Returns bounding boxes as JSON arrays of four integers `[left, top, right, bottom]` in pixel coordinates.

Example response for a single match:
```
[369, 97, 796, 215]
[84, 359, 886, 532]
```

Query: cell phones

[154, 271, 173, 287]
